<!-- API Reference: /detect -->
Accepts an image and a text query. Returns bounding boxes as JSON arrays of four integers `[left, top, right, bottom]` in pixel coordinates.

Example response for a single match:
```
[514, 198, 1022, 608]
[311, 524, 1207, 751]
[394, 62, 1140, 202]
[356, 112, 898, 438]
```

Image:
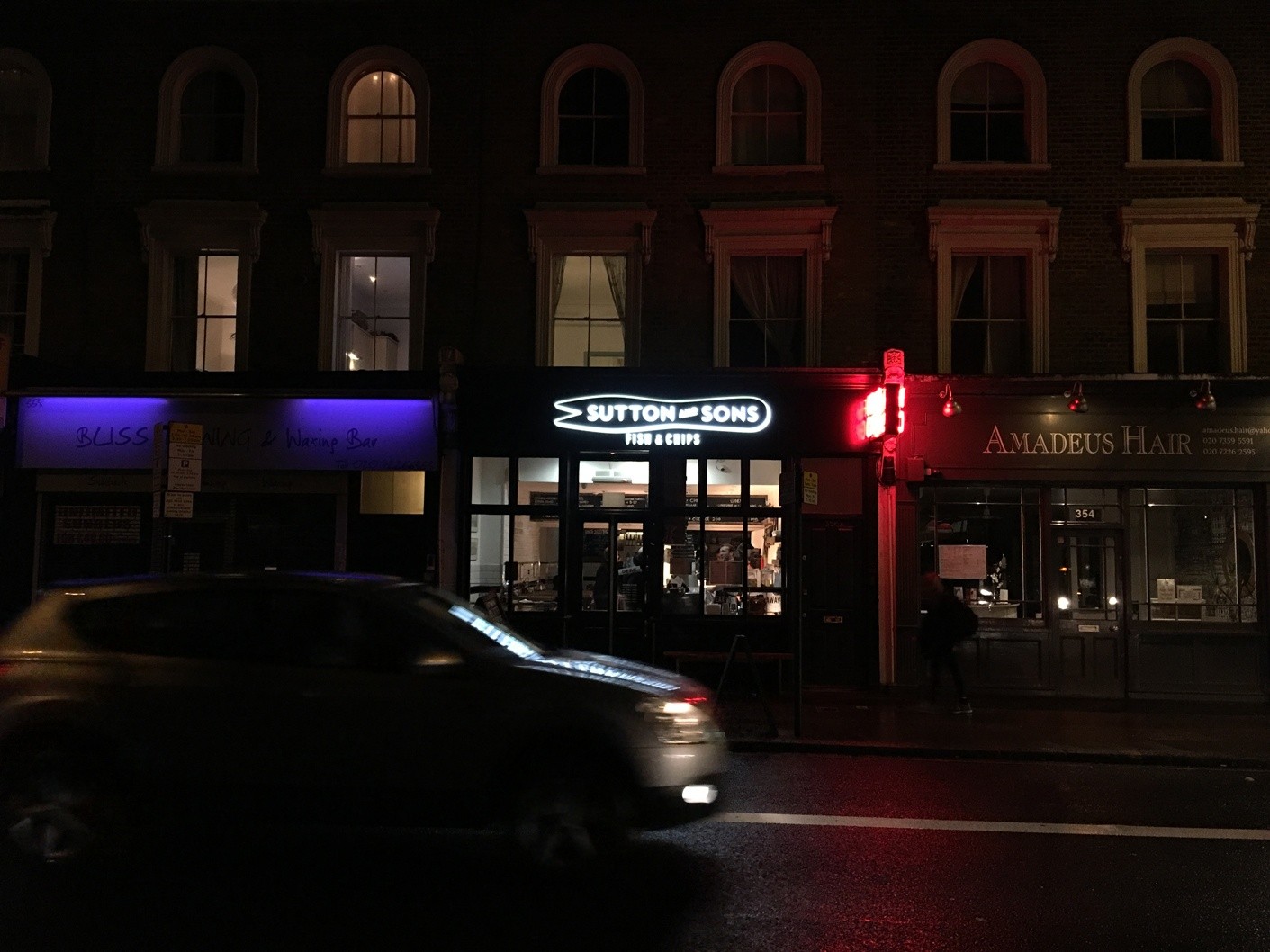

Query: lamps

[940, 382, 962, 417]
[1189, 376, 1217, 413]
[1063, 379, 1089, 413]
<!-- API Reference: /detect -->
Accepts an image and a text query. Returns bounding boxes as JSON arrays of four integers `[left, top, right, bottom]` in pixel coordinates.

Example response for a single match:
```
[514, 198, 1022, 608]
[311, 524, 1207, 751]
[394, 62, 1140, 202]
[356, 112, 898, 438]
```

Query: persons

[586, 584, 591, 591]
[714, 543, 735, 561]
[919, 561, 976, 716]
[589, 546, 610, 611]
[616, 533, 644, 611]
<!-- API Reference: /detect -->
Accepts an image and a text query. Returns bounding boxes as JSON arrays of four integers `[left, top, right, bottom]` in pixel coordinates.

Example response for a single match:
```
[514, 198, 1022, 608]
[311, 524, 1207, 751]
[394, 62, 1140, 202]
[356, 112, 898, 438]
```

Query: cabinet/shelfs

[347, 322, 398, 370]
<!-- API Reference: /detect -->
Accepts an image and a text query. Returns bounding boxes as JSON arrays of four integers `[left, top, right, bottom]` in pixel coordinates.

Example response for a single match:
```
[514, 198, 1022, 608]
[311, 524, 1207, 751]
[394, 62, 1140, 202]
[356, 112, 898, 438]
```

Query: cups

[748, 569, 758, 587]
[528, 587, 534, 598]
[760, 570, 774, 587]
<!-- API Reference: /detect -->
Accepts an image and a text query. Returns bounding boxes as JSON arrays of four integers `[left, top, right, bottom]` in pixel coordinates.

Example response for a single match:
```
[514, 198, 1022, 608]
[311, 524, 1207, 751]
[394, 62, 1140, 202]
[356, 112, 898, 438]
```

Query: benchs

[663, 651, 796, 701]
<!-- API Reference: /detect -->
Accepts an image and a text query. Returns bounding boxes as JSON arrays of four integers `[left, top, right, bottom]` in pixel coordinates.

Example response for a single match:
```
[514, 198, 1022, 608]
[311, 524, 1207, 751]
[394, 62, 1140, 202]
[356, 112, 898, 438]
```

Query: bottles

[626, 534, 643, 546]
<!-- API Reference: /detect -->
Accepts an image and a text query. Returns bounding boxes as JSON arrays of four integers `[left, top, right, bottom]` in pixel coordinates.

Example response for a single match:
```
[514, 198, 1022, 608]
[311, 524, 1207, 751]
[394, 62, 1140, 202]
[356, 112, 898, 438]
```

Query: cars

[0, 570, 732, 892]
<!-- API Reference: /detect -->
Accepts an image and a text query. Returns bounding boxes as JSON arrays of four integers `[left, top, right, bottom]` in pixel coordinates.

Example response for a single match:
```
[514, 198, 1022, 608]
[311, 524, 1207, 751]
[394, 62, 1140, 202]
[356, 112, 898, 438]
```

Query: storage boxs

[351, 310, 370, 329]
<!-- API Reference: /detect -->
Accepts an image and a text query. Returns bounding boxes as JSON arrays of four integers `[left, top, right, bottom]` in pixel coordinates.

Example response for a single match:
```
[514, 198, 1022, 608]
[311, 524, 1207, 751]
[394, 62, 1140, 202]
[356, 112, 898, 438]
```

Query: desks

[1149, 598, 1208, 621]
